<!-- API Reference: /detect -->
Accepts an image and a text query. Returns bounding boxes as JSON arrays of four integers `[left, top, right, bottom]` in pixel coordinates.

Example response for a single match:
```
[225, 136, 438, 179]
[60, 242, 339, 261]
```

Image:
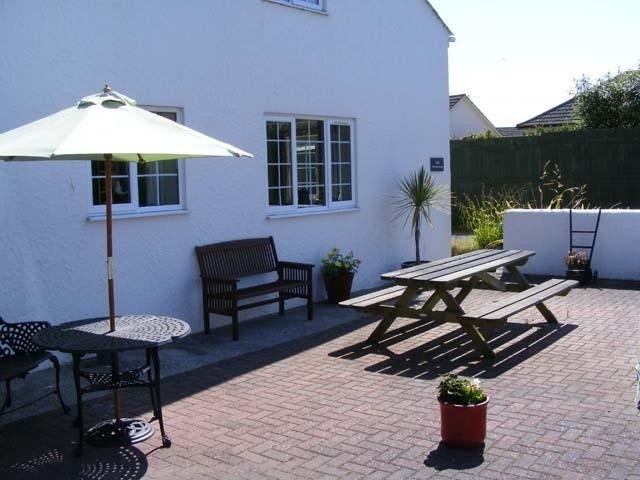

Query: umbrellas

[1, 82, 254, 421]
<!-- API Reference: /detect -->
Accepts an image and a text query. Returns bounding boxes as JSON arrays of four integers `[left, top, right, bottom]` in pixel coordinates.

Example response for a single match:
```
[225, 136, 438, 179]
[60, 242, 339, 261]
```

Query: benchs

[337, 284, 424, 314]
[195, 235, 316, 341]
[459, 278, 577, 326]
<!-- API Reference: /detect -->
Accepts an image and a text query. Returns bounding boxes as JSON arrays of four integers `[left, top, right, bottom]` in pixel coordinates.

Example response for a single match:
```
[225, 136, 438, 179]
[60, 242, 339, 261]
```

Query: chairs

[0, 314, 69, 413]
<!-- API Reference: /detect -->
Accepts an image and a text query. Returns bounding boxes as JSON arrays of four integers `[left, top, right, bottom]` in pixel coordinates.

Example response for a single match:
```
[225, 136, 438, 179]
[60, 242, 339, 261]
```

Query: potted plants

[380, 165, 457, 268]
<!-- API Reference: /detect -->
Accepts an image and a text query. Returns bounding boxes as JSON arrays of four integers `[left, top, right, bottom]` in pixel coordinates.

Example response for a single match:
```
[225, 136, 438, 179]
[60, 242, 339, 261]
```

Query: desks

[368, 248, 561, 360]
[32, 315, 191, 453]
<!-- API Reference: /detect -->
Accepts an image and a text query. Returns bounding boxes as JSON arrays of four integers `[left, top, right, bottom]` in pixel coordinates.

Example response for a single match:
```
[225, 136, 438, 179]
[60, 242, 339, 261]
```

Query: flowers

[564, 250, 590, 268]
[436, 372, 486, 404]
[319, 245, 360, 274]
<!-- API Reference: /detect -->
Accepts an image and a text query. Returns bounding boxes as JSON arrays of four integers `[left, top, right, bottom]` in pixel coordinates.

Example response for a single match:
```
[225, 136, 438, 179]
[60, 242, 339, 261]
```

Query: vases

[322, 273, 355, 304]
[439, 396, 489, 447]
[566, 268, 592, 285]
